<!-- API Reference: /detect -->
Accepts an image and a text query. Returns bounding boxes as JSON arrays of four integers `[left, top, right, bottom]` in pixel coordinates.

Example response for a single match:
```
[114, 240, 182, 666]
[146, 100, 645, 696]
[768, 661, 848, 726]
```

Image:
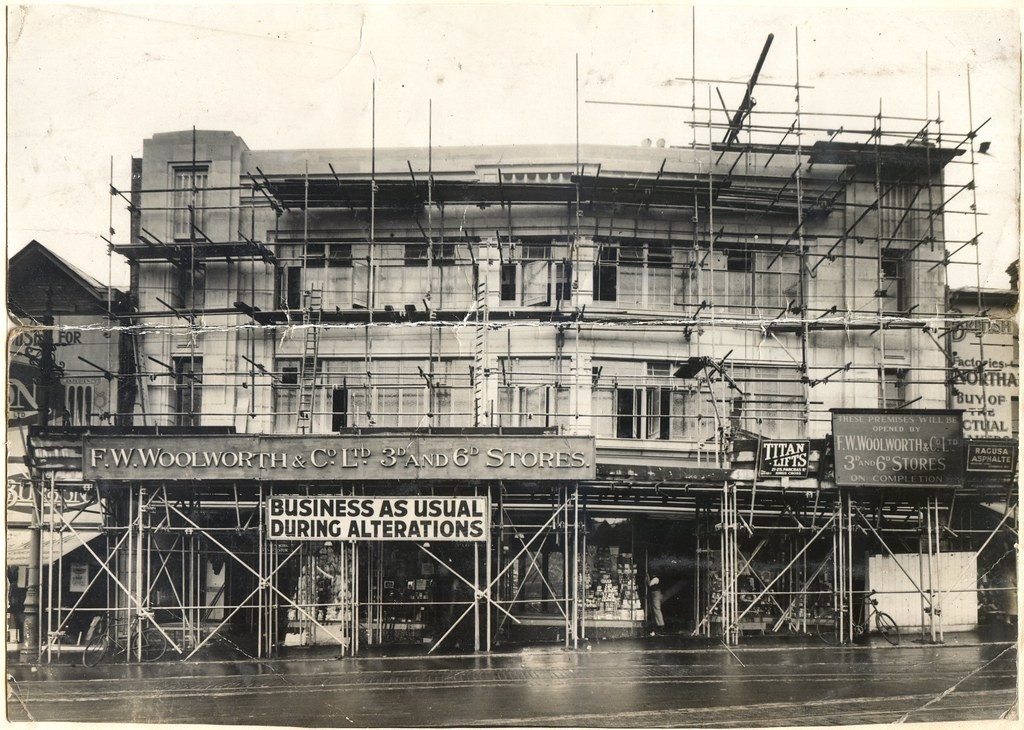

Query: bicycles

[82, 614, 167, 668]
[814, 589, 901, 645]
